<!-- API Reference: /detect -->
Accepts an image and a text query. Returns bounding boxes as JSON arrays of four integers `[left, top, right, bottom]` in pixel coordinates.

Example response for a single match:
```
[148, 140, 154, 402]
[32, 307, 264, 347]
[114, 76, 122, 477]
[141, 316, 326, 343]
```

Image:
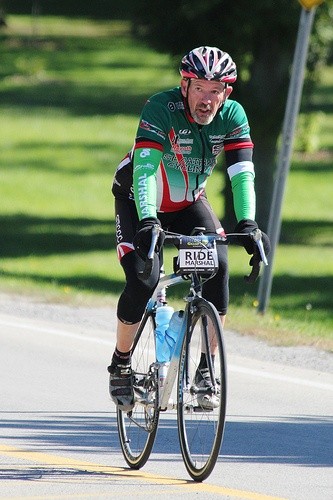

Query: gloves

[133, 217, 166, 263]
[234, 219, 271, 266]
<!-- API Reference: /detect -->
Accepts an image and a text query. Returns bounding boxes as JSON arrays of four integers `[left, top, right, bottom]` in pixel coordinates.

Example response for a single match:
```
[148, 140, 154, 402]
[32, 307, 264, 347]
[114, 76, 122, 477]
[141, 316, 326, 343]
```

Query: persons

[107, 46, 273, 413]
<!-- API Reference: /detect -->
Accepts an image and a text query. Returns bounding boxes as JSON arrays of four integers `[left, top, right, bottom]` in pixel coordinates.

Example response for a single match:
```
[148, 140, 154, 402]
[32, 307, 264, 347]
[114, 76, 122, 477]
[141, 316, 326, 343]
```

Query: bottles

[162, 309, 185, 360]
[155, 301, 174, 362]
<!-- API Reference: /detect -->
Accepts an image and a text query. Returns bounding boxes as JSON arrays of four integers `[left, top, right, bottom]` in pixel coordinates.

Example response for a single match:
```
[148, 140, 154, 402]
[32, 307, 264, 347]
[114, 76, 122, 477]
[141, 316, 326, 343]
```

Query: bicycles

[116, 225, 269, 480]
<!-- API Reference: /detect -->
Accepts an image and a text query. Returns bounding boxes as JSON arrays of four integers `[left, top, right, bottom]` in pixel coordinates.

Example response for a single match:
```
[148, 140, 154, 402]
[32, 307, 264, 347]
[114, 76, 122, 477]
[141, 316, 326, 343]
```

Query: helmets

[180, 47, 238, 82]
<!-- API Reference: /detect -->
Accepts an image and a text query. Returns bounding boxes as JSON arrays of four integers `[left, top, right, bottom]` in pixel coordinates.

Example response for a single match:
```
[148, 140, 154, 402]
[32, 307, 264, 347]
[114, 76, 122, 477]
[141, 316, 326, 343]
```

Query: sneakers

[108, 359, 136, 411]
[195, 366, 221, 409]
